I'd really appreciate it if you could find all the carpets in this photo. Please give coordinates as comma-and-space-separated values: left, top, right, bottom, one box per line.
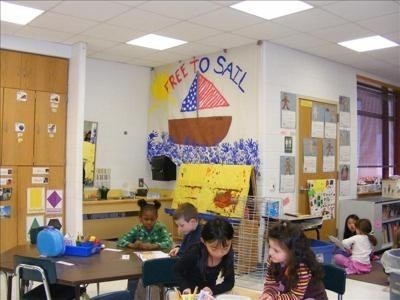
344, 252, 389, 287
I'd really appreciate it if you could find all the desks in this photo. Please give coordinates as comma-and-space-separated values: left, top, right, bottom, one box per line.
262, 213, 323, 240
0, 242, 178, 300
207, 283, 263, 300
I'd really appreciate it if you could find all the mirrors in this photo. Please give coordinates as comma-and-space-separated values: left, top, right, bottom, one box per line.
82, 120, 98, 187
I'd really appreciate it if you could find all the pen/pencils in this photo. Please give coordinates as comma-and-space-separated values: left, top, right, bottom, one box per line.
192, 286, 198, 300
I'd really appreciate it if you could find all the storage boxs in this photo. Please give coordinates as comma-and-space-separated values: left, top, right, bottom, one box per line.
309, 238, 335, 264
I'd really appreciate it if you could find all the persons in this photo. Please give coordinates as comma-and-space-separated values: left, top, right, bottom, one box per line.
171, 216, 235, 300
168, 203, 205, 257
260, 218, 329, 300
116, 198, 173, 253
343, 214, 380, 261
329, 219, 378, 275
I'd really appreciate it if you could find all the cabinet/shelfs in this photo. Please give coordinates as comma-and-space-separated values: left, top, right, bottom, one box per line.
336, 196, 400, 253
82, 196, 173, 245
0, 48, 69, 273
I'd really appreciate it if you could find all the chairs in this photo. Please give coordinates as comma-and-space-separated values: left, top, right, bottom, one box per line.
142, 257, 186, 300
11, 254, 87, 300
319, 263, 346, 300
30, 226, 60, 244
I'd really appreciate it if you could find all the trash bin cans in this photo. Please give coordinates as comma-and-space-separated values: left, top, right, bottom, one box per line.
385, 249, 400, 300
